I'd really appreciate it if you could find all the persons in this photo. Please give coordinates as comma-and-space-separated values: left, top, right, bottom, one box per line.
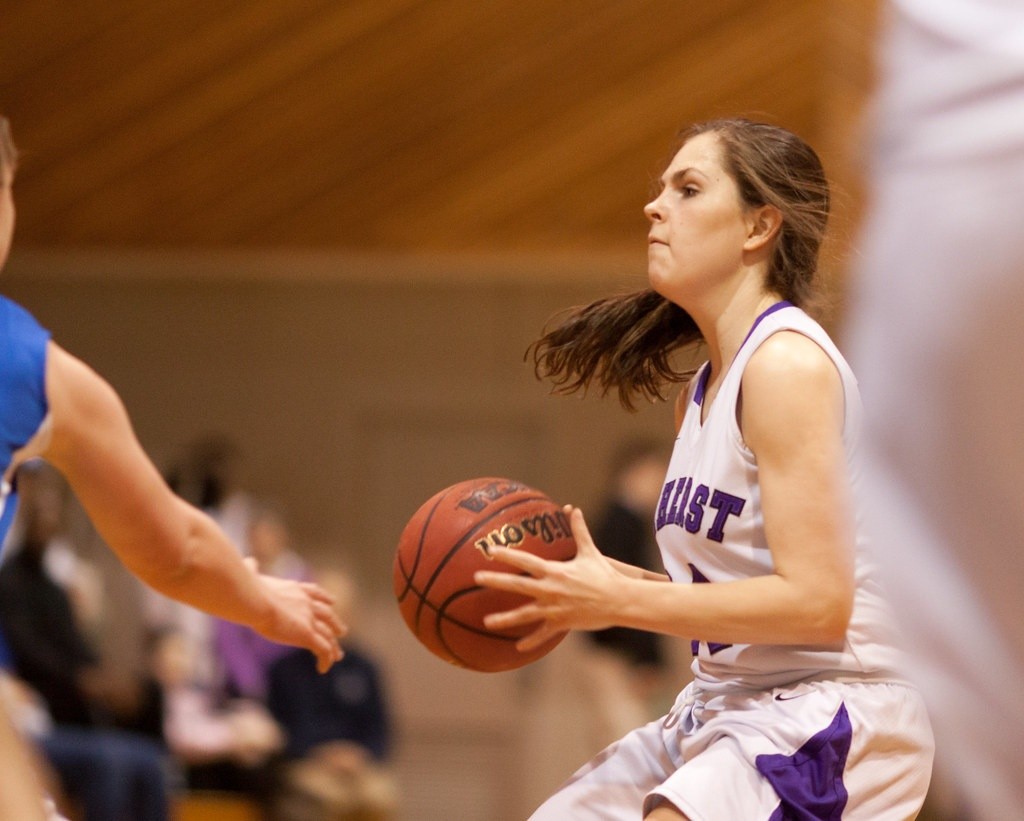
0, 111, 347, 821
0, 431, 400, 821
472, 116, 935, 821
573, 432, 672, 741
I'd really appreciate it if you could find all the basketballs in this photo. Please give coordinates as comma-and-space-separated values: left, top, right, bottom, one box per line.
392, 478, 577, 678
